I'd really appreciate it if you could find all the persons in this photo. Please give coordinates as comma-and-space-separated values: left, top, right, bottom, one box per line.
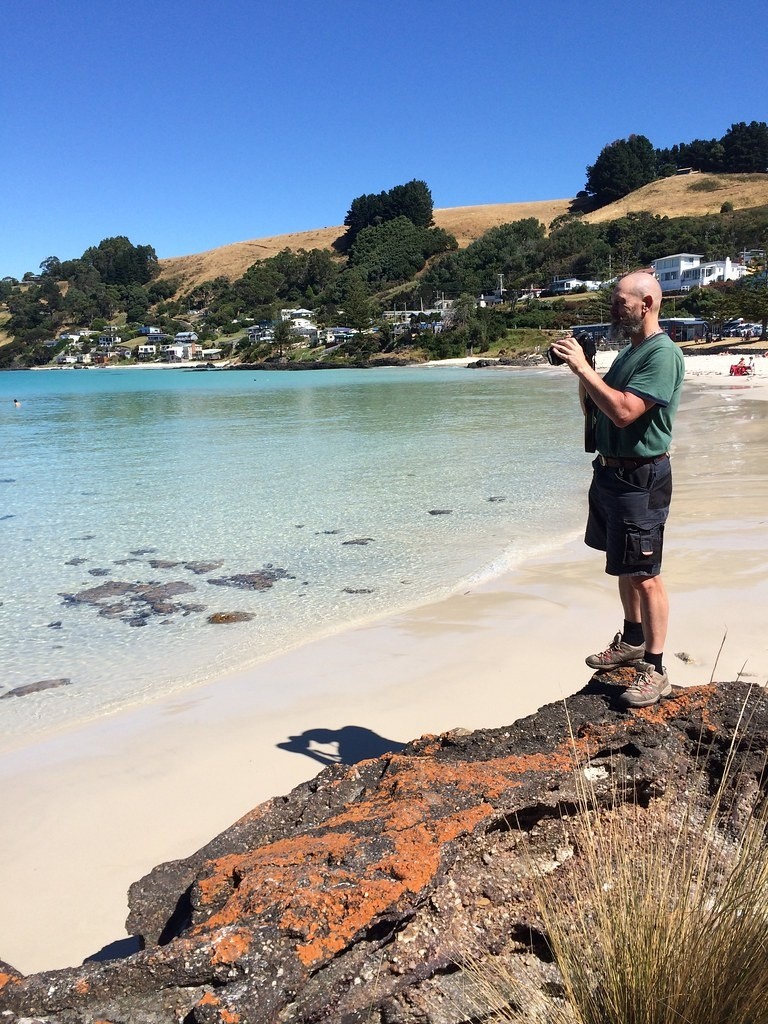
551, 273, 685, 707
730, 357, 754, 376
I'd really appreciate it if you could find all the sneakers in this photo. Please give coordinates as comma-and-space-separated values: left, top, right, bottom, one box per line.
585, 629, 647, 670
621, 661, 672, 707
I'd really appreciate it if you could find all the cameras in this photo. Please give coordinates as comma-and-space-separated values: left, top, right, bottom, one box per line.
547, 329, 597, 367
